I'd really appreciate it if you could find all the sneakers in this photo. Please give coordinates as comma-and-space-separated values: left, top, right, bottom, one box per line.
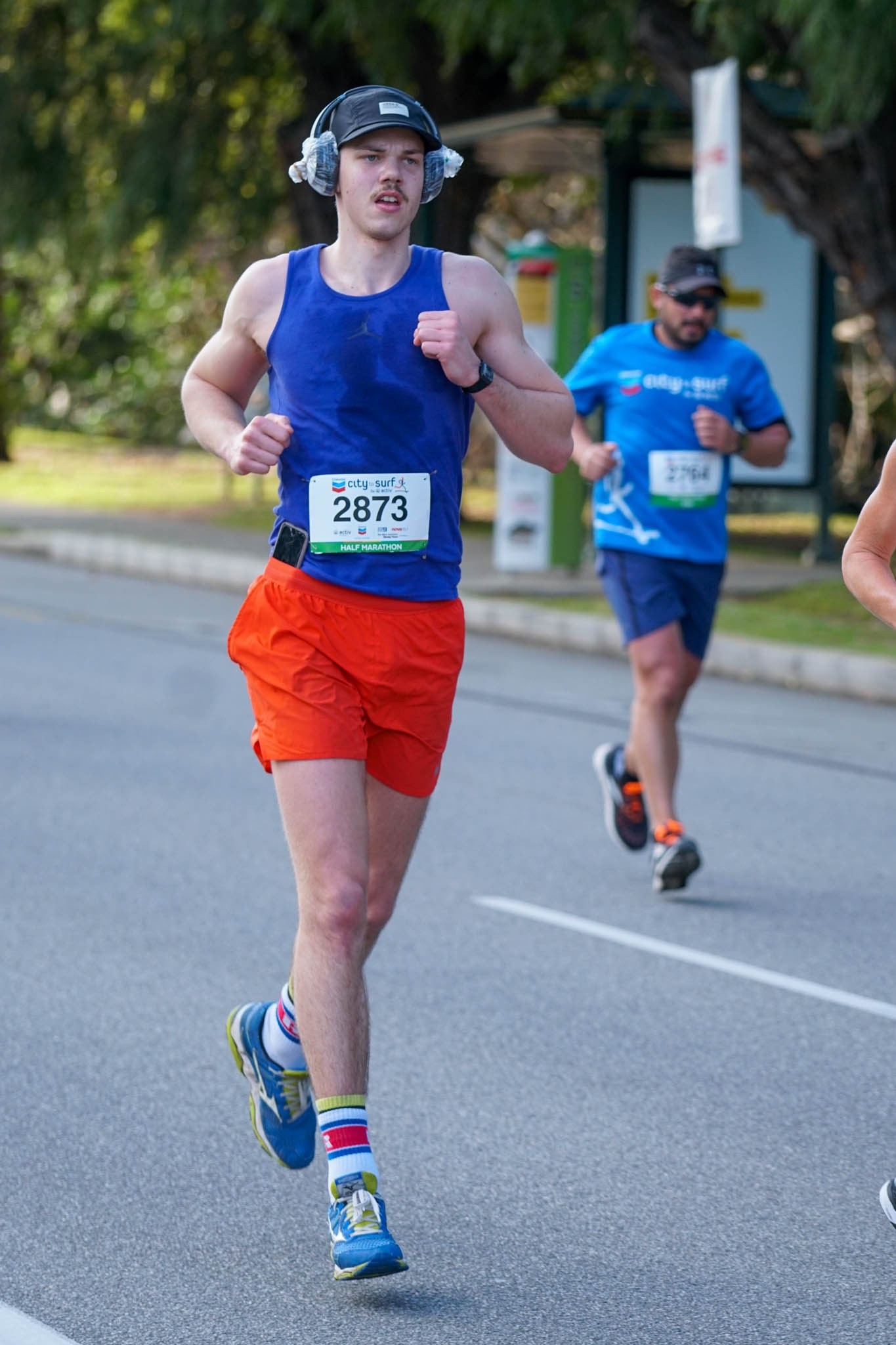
594, 740, 648, 854
647, 819, 700, 895
224, 997, 318, 1170
326, 1170, 413, 1283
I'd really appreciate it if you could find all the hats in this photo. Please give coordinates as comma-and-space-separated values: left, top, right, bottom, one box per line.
328, 89, 442, 156
661, 244, 731, 298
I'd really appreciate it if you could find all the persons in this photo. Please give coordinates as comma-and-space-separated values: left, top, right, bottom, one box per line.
842, 439, 896, 629
562, 243, 792, 893
179, 85, 578, 1286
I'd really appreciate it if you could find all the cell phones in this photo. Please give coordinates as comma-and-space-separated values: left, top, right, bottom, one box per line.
272, 521, 308, 569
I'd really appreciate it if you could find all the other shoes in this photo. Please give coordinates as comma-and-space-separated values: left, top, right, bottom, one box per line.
879, 1177, 896, 1227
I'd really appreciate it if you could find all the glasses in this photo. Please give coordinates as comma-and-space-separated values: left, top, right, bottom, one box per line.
673, 292, 717, 310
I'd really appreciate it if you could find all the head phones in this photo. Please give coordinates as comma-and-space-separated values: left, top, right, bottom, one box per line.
288, 85, 464, 204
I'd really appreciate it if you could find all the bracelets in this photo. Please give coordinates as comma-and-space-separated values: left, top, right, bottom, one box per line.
735, 432, 749, 454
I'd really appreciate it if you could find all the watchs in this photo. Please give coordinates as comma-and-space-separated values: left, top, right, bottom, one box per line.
460, 357, 494, 393
